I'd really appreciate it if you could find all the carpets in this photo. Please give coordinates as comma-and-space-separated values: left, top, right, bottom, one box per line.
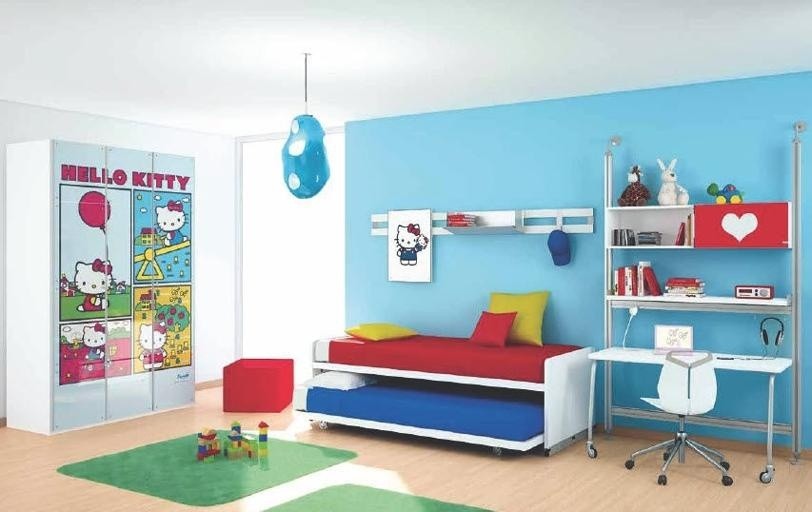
54, 424, 356, 508
260, 483, 501, 512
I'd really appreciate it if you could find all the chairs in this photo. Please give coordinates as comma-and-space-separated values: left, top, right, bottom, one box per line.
626, 348, 739, 491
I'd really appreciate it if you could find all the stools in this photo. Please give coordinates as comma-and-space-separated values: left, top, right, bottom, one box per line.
223, 359, 294, 413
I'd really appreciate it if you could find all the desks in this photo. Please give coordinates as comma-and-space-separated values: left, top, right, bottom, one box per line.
582, 344, 794, 487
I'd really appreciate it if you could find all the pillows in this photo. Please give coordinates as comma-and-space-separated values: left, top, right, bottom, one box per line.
344, 322, 422, 342
305, 373, 381, 391
489, 289, 551, 348
468, 309, 517, 347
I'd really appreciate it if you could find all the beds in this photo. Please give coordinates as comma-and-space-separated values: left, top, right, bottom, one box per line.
303, 332, 597, 459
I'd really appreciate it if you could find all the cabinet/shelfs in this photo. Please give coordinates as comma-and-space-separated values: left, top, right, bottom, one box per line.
4, 136, 201, 440
600, 115, 807, 464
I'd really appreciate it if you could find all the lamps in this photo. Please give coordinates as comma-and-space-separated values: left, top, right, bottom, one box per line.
621, 302, 642, 353
276, 49, 333, 199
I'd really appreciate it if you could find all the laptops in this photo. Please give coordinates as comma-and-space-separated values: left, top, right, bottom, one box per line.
654, 324, 693, 357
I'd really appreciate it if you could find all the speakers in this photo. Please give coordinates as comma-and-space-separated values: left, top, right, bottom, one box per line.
735, 285, 775, 299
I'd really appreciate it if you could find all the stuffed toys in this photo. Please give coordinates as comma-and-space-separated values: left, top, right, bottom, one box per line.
657, 156, 689, 205
616, 163, 653, 207
705, 181, 745, 205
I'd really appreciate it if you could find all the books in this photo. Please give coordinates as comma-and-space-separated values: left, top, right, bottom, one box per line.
612, 263, 705, 299
446, 213, 481, 229
674, 221, 685, 245
684, 214, 692, 246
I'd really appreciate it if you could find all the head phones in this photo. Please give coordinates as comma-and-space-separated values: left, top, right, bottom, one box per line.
761, 317, 784, 346
